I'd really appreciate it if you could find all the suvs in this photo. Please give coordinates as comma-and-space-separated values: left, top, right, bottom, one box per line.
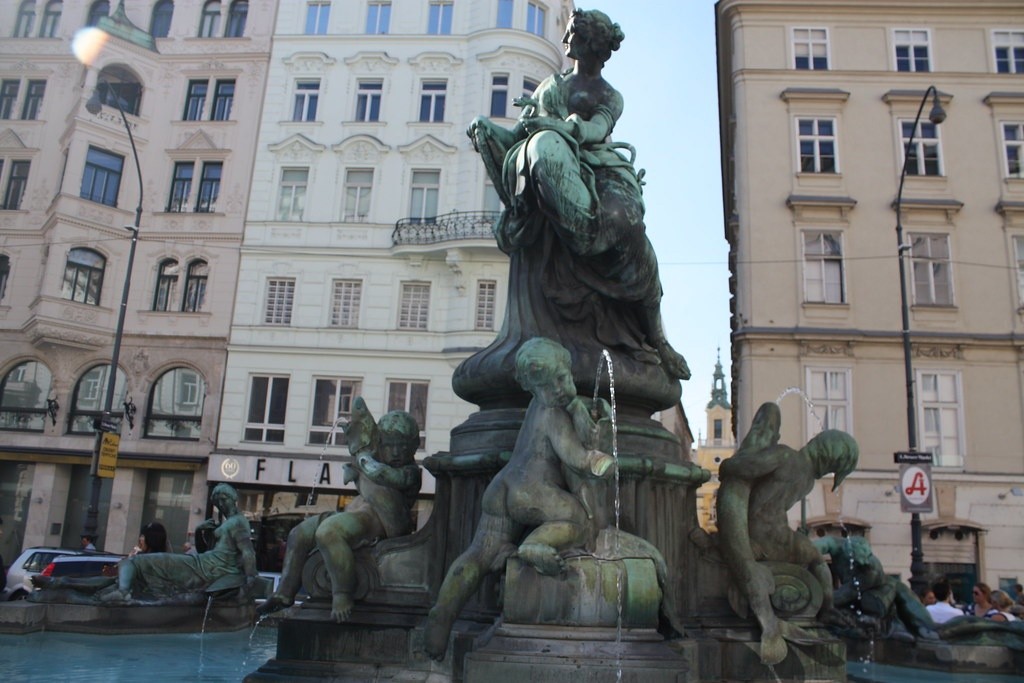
43, 555, 123, 580
1, 544, 117, 601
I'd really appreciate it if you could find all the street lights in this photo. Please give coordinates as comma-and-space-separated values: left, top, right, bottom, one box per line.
83, 72, 144, 559
894, 84, 949, 603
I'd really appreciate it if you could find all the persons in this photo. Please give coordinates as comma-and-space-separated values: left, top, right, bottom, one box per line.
135, 522, 167, 553
423, 337, 618, 659
950, 582, 998, 619
182, 542, 196, 555
1013, 584, 1024, 605
690, 428, 860, 628
467, 7, 692, 381
82, 537, 95, 549
255, 410, 422, 623
98, 485, 259, 606
920, 587, 937, 607
925, 581, 964, 623
990, 590, 1016, 621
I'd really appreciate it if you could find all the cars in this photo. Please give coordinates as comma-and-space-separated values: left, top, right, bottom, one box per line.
251, 568, 307, 609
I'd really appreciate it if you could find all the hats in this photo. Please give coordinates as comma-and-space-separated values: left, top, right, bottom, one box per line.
80, 533, 93, 539
